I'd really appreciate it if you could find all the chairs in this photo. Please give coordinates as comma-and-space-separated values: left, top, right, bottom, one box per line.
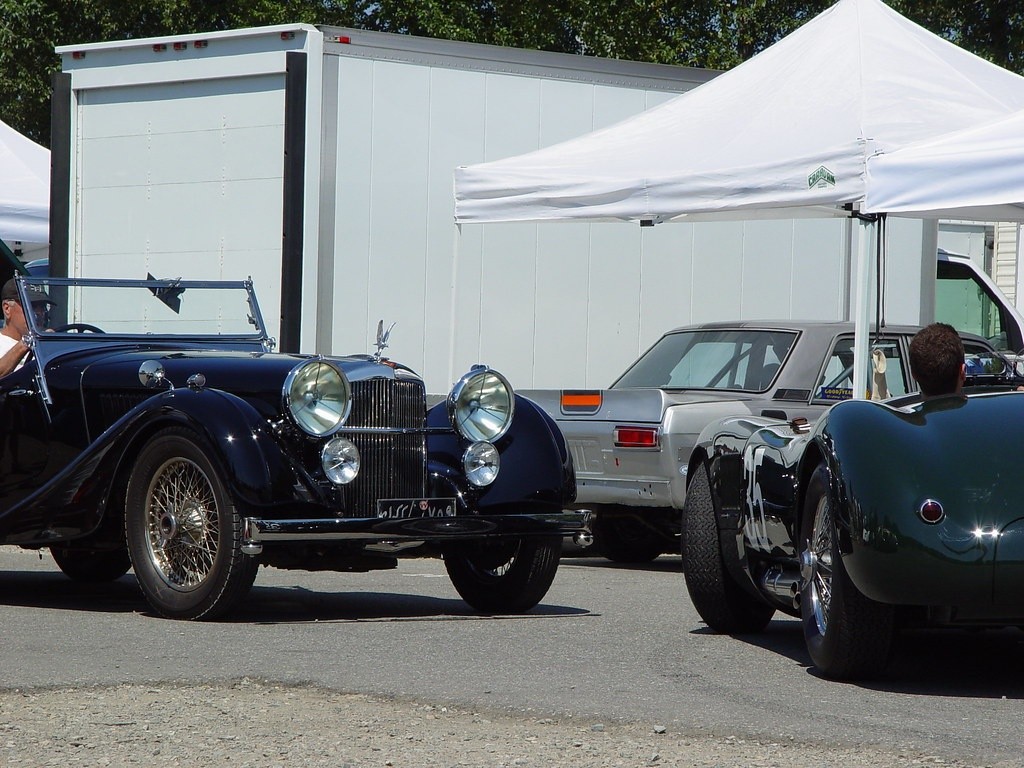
759, 364, 778, 389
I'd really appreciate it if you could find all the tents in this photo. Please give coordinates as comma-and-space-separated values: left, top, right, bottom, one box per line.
0, 120, 51, 260
450, 0, 1024, 403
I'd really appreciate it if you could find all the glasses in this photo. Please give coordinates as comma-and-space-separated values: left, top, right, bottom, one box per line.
7, 299, 55, 314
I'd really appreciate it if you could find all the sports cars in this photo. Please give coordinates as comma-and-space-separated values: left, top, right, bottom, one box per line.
678, 397, 1022, 682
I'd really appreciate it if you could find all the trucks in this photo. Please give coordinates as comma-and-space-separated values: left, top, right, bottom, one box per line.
50, 22, 1022, 394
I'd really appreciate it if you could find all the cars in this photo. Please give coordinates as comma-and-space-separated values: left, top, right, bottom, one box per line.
0, 267, 598, 625
515, 318, 1023, 567
23, 256, 49, 293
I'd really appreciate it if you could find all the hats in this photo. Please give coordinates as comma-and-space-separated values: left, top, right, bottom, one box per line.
2, 275, 58, 307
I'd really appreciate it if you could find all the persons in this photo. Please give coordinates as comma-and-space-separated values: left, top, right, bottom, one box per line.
910, 322, 966, 396
0, 279, 55, 380
